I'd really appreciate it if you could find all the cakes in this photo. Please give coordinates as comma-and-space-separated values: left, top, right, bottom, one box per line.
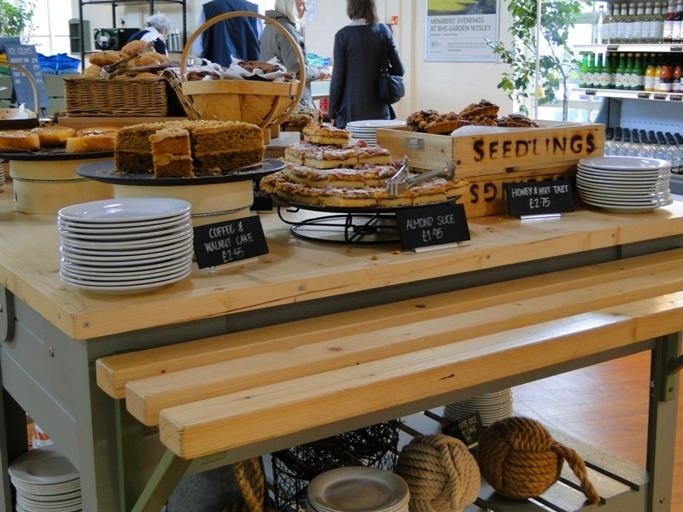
115, 121, 264, 175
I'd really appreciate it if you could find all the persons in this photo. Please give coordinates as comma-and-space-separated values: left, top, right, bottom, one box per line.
260, 0, 331, 111
327, 0, 404, 130
127, 13, 171, 57
196, 0, 262, 68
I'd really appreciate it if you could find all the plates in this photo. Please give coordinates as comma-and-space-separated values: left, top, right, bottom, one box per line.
4, 445, 82, 511
346, 119, 405, 147
0, 143, 110, 160
443, 390, 514, 428
0, 163, 5, 190
573, 154, 674, 214
54, 199, 195, 298
307, 466, 414, 511
73, 156, 288, 186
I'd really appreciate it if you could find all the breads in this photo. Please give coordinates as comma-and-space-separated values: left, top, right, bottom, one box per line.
82, 40, 291, 82
260, 124, 470, 203
407, 100, 539, 133
0, 124, 118, 158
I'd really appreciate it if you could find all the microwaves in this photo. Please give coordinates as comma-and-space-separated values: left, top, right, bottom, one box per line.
93, 27, 139, 50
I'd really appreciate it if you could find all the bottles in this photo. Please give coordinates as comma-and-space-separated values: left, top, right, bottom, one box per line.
578, 50, 683, 94
166, 28, 182, 52
604, 125, 683, 185
594, 3, 679, 43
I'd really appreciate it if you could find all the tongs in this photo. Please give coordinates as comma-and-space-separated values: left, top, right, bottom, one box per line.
387, 154, 456, 198
99, 52, 140, 80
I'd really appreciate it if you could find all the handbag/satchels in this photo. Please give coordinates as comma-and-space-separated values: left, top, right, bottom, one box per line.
374, 23, 403, 104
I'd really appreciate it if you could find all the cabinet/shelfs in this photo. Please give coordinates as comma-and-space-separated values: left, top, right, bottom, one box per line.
562, 2, 682, 201
77, 1, 189, 77
1, 125, 682, 512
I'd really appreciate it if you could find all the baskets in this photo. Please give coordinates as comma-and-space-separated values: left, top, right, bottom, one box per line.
179, 11, 304, 144
62, 76, 168, 118
